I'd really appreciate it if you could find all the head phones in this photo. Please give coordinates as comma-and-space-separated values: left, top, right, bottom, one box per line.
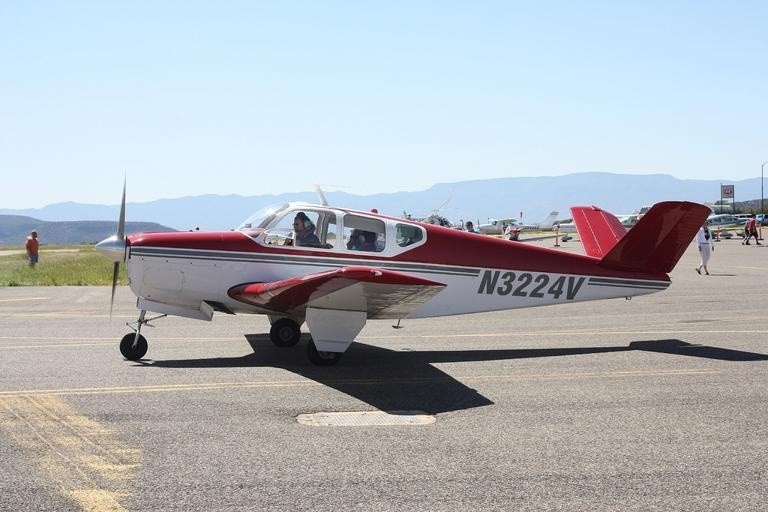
359, 234, 366, 244
296, 212, 311, 231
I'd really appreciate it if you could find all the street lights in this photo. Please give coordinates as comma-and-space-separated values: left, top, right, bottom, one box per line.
760, 159, 768, 227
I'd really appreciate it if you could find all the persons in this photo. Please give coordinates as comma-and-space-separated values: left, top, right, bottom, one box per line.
347, 228, 377, 252
466, 220, 481, 234
696, 219, 714, 275
742, 209, 762, 246
25, 229, 40, 269
284, 211, 321, 247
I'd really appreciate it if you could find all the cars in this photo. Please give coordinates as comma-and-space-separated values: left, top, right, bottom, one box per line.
755, 214, 768, 226
